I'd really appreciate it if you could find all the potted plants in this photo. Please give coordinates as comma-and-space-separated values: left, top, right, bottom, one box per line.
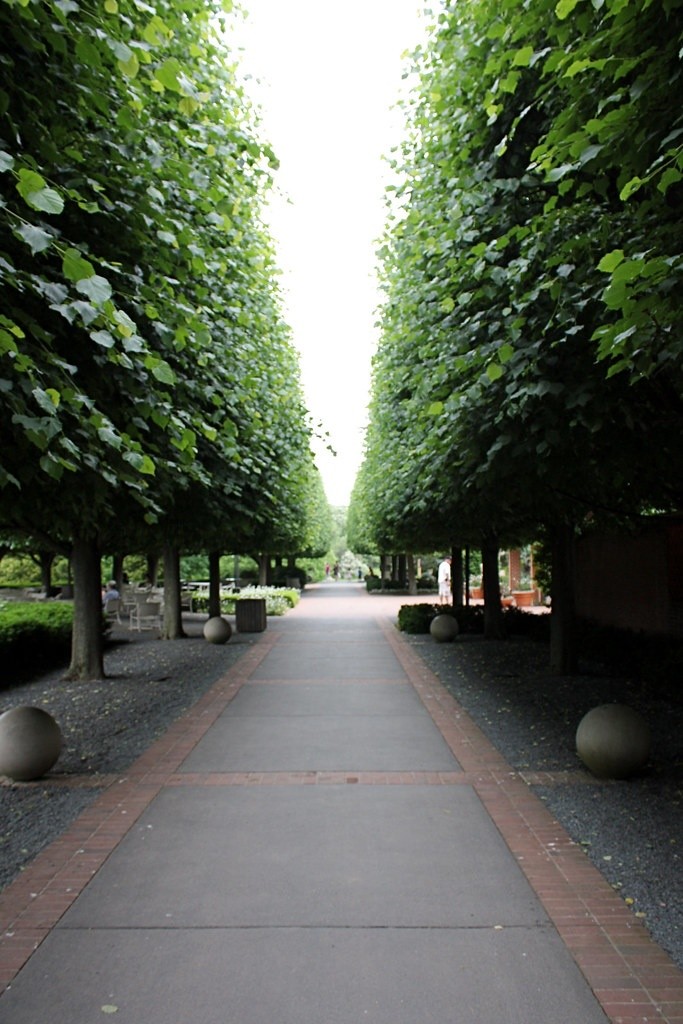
469, 574, 486, 600
511, 575, 540, 608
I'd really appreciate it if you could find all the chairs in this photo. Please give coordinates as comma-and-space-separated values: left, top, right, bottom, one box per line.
103, 584, 193, 633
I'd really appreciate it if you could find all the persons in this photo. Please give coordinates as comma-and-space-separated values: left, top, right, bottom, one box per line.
102, 581, 119, 608
334, 561, 341, 582
437, 556, 451, 604
369, 566, 373, 574
358, 566, 362, 579
345, 568, 352, 582
325, 562, 330, 578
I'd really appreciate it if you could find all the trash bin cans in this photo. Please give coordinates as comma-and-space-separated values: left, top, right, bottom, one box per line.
235, 598, 268, 633
